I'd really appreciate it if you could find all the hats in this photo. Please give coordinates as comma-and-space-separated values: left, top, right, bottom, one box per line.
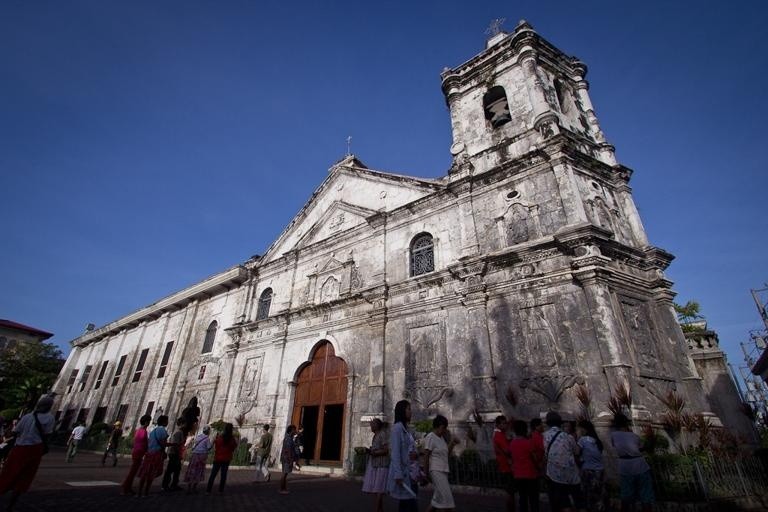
113, 420, 122, 426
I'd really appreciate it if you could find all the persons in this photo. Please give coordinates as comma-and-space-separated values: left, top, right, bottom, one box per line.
293, 425, 306, 471
0, 392, 55, 512
61, 419, 88, 464
204, 422, 237, 496
100, 420, 122, 468
251, 424, 274, 483
12, 418, 18, 430
159, 415, 188, 491
133, 414, 177, 500
354, 397, 457, 510
493, 410, 655, 511
183, 425, 217, 495
118, 414, 151, 496
276, 423, 298, 494
0, 418, 16, 465
